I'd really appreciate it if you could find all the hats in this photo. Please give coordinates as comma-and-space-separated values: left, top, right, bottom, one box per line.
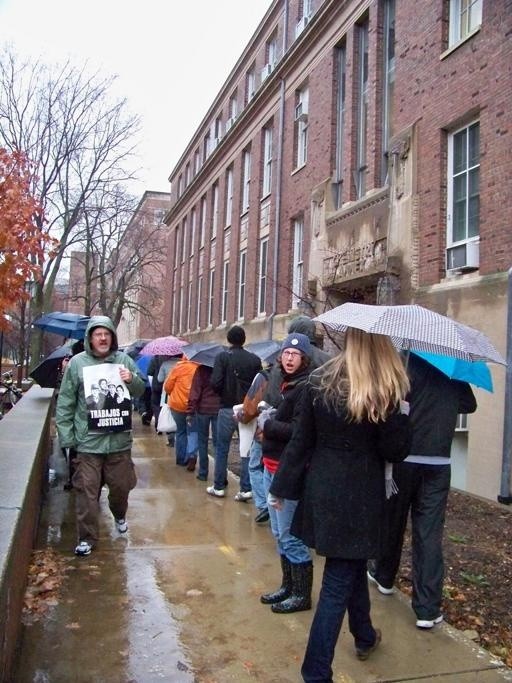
226, 325, 245, 345
280, 333, 312, 358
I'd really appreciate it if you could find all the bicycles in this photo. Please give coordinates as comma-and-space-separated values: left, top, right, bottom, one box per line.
0, 369, 22, 406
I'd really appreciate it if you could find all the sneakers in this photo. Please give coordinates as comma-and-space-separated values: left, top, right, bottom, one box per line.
166, 442, 175, 447
255, 507, 270, 523
415, 613, 444, 629
115, 518, 129, 534
206, 484, 225, 498
196, 474, 229, 488
74, 541, 94, 556
64, 480, 73, 490
234, 489, 253, 502
186, 456, 196, 472
367, 570, 393, 595
355, 629, 382, 662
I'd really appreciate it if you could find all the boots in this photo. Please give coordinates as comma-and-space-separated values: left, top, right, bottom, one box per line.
260, 553, 293, 604
271, 560, 314, 613
